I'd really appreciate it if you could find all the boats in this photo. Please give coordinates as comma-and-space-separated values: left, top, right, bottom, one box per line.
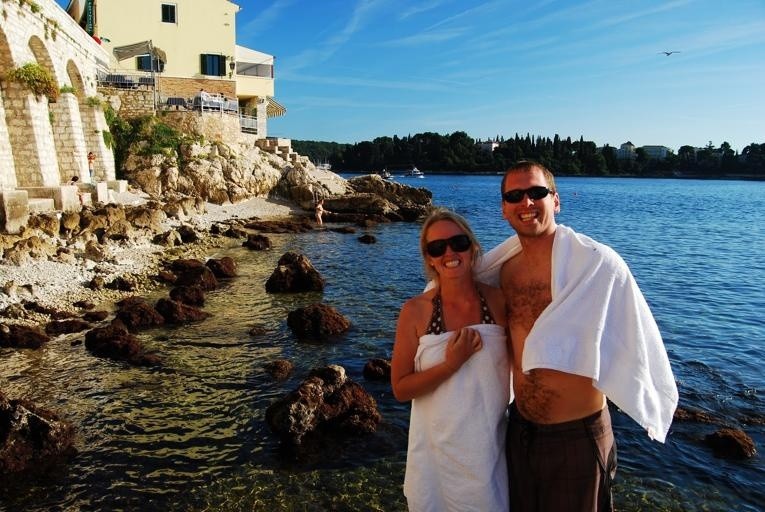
404, 167, 424, 179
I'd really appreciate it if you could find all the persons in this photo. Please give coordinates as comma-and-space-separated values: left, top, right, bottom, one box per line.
498, 161, 620, 511
390, 206, 507, 512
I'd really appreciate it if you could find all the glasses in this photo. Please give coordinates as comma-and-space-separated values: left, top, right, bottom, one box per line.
501, 185, 554, 204
426, 234, 471, 257
